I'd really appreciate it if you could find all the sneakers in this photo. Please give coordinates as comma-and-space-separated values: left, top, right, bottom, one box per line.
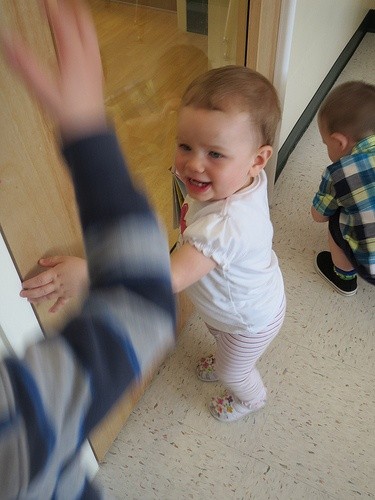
313, 248, 359, 296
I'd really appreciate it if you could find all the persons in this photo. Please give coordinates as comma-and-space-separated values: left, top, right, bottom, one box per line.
310, 80, 375, 296
0, 0, 175, 499
19, 64, 286, 423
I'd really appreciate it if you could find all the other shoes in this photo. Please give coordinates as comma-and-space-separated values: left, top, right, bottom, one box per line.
196, 351, 224, 382
208, 391, 266, 422
